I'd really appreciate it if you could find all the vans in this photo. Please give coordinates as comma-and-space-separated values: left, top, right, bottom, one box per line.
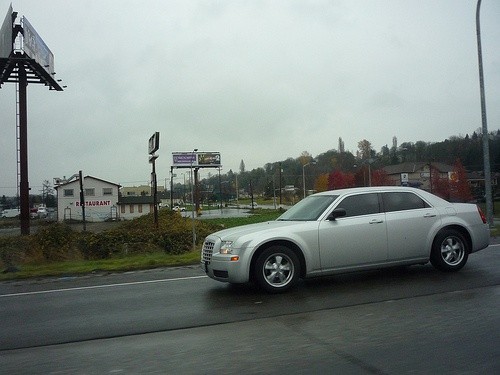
37, 207, 47, 213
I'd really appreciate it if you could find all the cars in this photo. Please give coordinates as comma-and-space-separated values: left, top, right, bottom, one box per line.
249, 202, 257, 206
200, 186, 492, 296
30, 213, 49, 220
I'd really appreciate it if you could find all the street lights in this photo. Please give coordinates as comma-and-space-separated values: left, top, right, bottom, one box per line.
303, 161, 317, 198
353, 164, 365, 186
368, 147, 409, 187
191, 148, 198, 252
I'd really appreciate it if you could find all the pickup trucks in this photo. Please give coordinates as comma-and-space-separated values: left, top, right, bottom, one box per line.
172, 206, 185, 212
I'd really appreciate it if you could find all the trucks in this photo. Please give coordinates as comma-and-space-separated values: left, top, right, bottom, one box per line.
1, 208, 20, 220
